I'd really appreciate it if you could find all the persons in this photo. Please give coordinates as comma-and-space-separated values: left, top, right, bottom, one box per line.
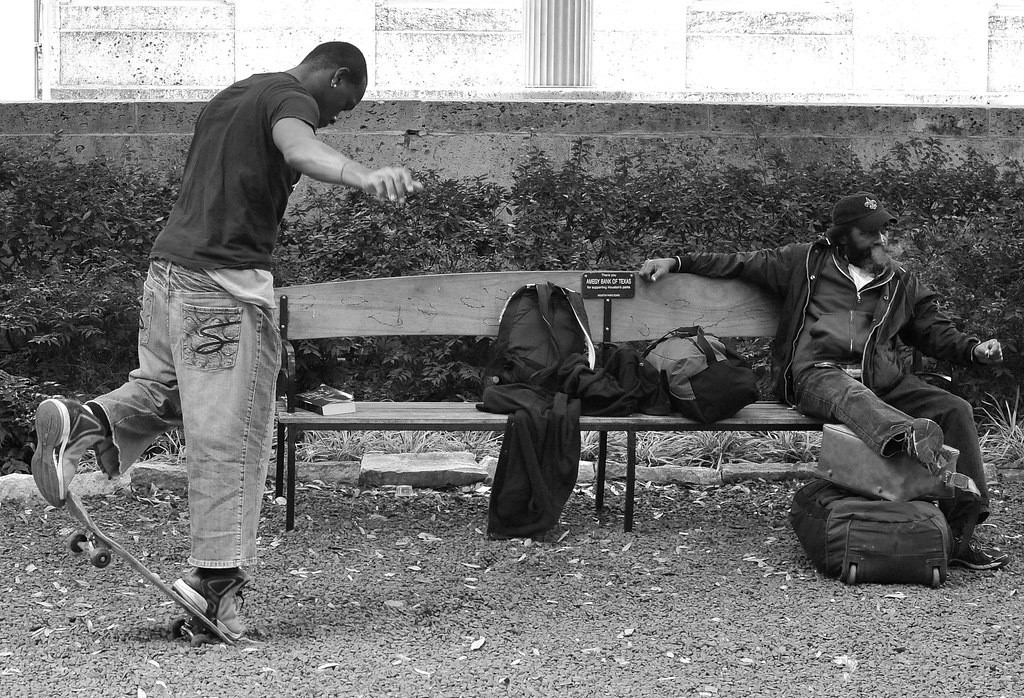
639, 191, 1010, 570
31, 42, 423, 640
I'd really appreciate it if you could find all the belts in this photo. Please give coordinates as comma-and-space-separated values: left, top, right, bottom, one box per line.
154, 257, 208, 277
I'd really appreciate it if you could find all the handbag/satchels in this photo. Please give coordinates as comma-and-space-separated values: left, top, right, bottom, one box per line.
814, 423, 983, 502
642, 325, 760, 425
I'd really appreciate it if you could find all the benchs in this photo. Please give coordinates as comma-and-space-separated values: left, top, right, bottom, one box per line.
275, 271, 838, 534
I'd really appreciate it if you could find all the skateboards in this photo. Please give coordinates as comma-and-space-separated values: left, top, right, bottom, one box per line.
64, 493, 240, 647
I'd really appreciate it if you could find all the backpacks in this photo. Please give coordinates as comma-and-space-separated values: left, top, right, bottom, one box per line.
483, 282, 595, 395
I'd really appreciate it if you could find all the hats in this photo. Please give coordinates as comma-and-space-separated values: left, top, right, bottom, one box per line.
833, 191, 898, 235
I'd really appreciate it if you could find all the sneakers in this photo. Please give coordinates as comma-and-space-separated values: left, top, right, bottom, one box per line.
890, 418, 952, 474
31, 399, 107, 507
947, 536, 1012, 570
172, 566, 251, 641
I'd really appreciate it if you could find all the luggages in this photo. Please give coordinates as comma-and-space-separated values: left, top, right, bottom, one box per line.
789, 478, 950, 588
